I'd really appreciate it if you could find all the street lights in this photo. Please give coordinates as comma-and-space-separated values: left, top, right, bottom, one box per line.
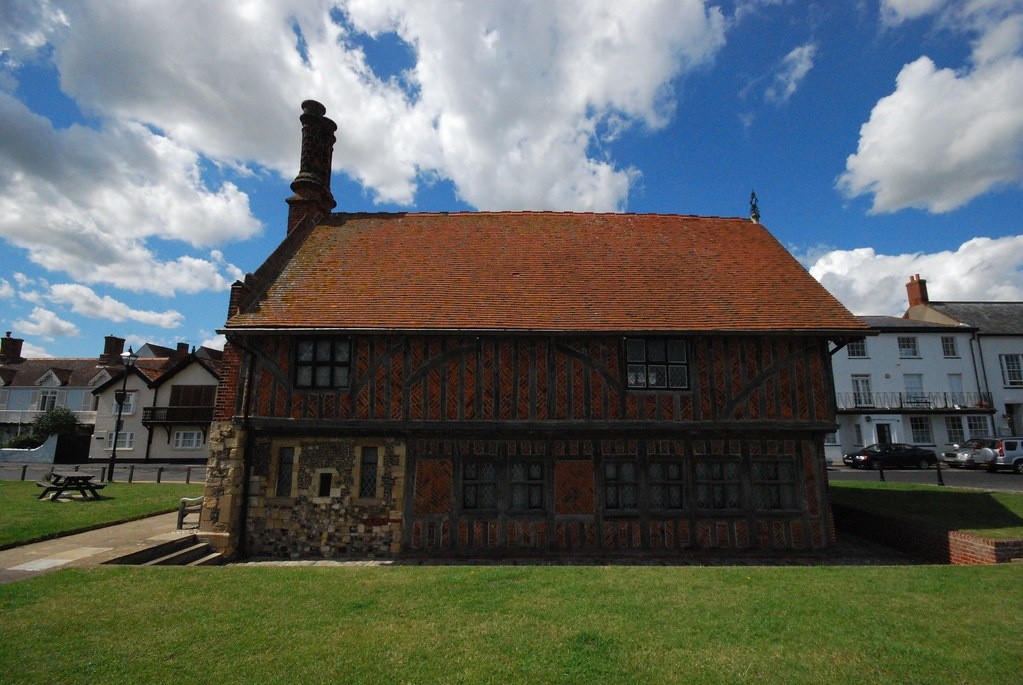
107, 344, 139, 482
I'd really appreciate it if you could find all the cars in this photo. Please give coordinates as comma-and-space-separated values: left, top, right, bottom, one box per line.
842, 442, 939, 471
941, 437, 994, 469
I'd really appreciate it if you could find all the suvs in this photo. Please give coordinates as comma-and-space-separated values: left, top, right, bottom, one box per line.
979, 436, 1023, 475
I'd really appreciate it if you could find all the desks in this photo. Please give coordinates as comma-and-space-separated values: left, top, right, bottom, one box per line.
39, 471, 100, 502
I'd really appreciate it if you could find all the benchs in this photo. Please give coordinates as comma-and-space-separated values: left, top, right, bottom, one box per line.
35, 481, 106, 501
177, 495, 205, 530
